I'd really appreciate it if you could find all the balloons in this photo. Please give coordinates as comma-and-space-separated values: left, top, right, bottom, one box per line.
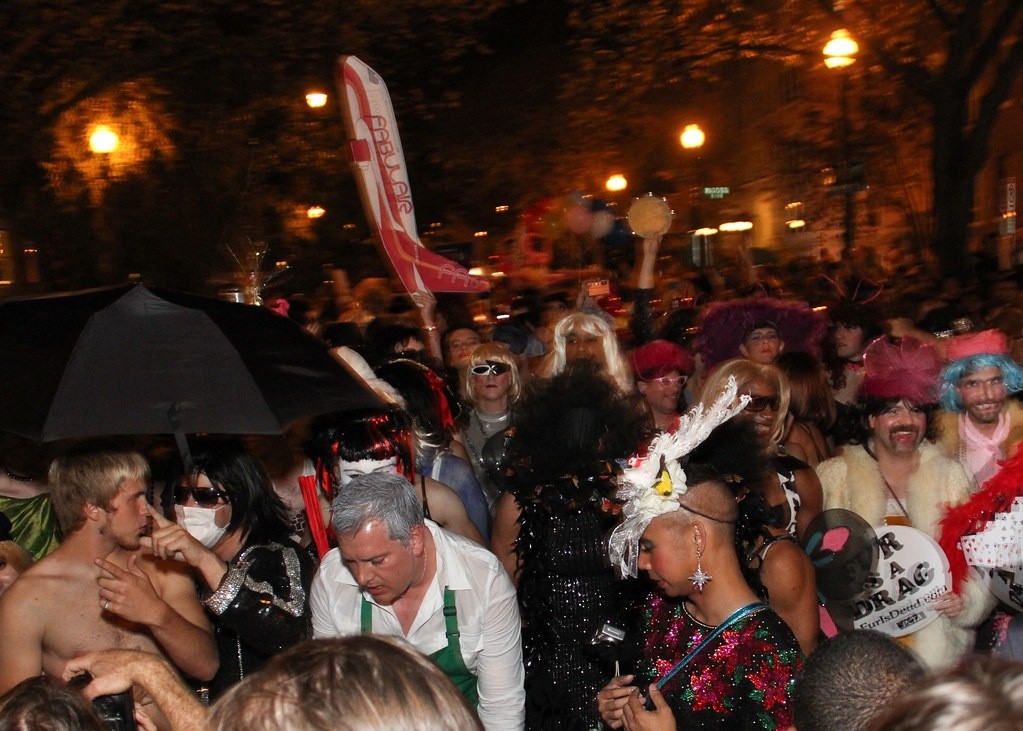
517, 191, 614, 262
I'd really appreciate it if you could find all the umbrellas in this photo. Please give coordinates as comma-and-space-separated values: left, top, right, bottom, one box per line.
0, 274, 392, 450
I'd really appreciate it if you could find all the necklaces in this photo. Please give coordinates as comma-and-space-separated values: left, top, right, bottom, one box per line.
0, 461, 34, 481
845, 359, 864, 371
409, 548, 427, 587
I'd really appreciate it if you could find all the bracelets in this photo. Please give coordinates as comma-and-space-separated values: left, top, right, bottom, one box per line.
422, 324, 439, 331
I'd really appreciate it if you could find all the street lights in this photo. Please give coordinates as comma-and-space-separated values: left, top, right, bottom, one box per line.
678, 121, 708, 302
822, 27, 859, 262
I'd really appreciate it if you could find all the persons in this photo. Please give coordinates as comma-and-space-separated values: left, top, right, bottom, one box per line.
0, 237, 1023, 731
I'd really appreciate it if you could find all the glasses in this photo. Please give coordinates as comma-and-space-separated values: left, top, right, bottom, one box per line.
650, 376, 688, 387
735, 393, 780, 413
470, 365, 511, 375
173, 487, 228, 508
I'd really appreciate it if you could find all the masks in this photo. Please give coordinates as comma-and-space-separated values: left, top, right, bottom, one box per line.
172, 503, 234, 550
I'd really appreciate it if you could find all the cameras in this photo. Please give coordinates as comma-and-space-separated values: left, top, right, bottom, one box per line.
590, 623, 625, 653
67, 674, 138, 731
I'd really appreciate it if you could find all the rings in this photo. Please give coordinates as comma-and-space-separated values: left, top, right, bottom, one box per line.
103, 601, 110, 608
